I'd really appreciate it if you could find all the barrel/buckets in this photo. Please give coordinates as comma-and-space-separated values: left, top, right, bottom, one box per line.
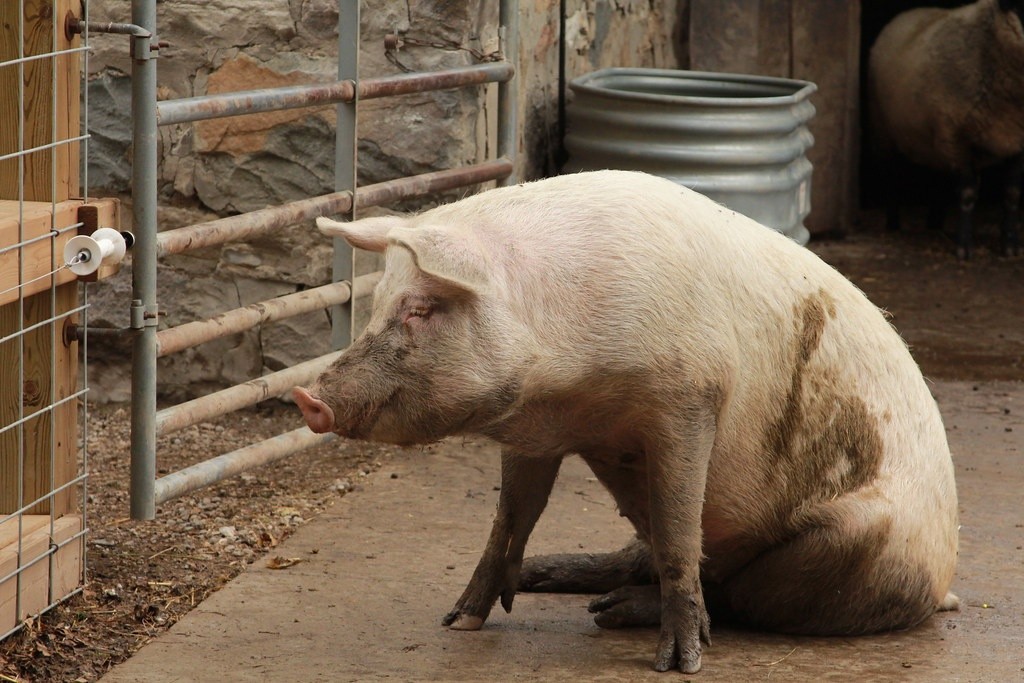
566, 67, 817, 249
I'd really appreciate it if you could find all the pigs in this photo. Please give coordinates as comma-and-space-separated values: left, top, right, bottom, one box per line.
289, 169, 963, 675
867, 0, 1024, 210
